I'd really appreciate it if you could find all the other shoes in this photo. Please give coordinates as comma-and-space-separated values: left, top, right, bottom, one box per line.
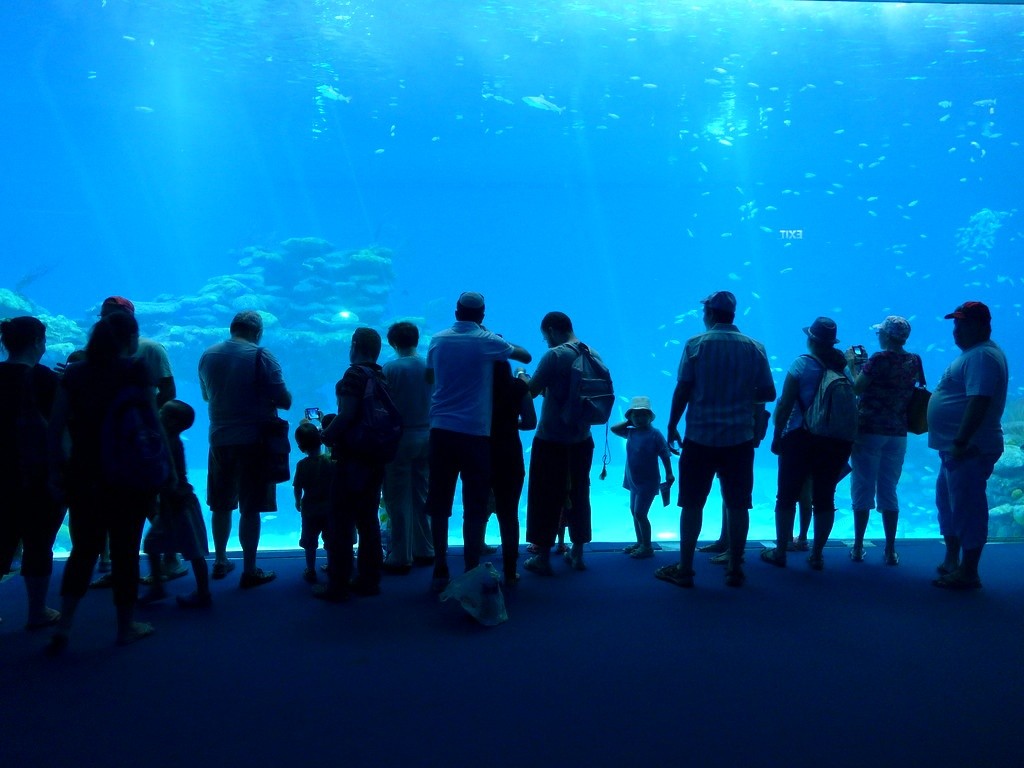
807, 552, 824, 572
761, 548, 786, 567
882, 548, 900, 565
934, 569, 983, 589
936, 559, 961, 574
850, 547, 866, 562
785, 537, 809, 552
27, 539, 748, 650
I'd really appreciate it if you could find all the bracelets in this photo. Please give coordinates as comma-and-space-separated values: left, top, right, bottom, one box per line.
515, 371, 525, 378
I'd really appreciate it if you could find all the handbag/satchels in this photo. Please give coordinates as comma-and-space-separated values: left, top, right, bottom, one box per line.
253, 415, 291, 484
907, 354, 932, 435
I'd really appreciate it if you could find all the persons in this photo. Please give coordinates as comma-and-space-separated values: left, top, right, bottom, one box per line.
293, 329, 387, 598
381, 323, 435, 574
0, 316, 69, 632
844, 316, 931, 565
517, 312, 594, 574
655, 292, 853, 588
611, 407, 674, 558
423, 292, 536, 592
927, 301, 1009, 587
198, 312, 291, 590
56, 297, 214, 640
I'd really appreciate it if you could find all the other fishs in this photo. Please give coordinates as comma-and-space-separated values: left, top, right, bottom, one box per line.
83, 0, 1024, 527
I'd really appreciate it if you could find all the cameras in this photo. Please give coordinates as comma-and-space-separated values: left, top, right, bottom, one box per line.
852, 345, 863, 357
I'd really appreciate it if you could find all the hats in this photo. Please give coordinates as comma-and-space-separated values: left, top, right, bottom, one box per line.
625, 397, 656, 423
700, 290, 737, 313
458, 291, 484, 309
802, 317, 841, 344
102, 295, 134, 317
873, 316, 911, 341
945, 301, 991, 324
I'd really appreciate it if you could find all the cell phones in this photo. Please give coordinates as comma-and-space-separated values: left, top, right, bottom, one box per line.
306, 408, 320, 420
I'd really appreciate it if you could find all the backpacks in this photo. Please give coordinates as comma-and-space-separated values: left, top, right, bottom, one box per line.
795, 354, 859, 447
546, 342, 615, 426
353, 365, 405, 450
100, 356, 177, 495
